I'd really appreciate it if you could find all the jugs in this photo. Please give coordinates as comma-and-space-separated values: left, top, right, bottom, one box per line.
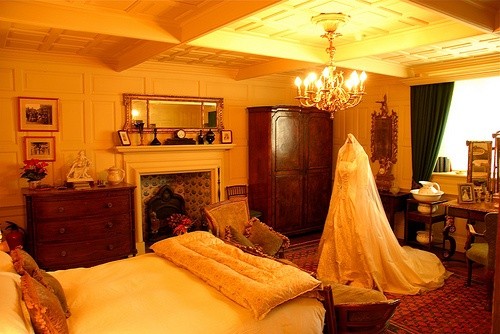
106, 166, 125, 184
418, 181, 440, 195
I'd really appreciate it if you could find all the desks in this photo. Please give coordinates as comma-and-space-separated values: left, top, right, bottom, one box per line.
377, 192, 411, 246
440, 199, 500, 265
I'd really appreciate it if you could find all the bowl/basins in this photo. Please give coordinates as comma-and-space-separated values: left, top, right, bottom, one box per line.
410, 189, 444, 201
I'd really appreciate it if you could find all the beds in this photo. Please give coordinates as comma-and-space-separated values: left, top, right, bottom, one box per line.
0, 245, 337, 334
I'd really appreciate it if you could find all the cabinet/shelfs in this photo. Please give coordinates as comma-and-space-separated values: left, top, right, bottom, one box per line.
404, 198, 448, 256
246, 103, 333, 237
22, 184, 138, 271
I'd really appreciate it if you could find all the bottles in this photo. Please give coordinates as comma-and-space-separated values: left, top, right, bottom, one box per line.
416, 231, 433, 242
151, 128, 161, 145
198, 130, 204, 144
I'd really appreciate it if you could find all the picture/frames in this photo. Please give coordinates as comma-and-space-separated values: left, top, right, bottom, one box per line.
457, 182, 475, 204
117, 130, 132, 146
221, 129, 233, 144
23, 135, 57, 162
17, 96, 60, 132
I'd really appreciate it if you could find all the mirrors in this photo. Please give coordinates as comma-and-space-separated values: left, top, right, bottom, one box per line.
121, 93, 226, 131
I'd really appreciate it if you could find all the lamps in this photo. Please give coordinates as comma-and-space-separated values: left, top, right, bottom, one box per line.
292, 11, 369, 118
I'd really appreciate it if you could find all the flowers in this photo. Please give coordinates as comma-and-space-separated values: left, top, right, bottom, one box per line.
18, 158, 49, 180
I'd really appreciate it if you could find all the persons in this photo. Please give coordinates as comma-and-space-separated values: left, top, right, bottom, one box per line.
316, 134, 455, 294
28, 112, 47, 121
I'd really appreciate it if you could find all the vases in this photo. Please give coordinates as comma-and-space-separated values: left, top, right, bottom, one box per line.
29, 179, 43, 190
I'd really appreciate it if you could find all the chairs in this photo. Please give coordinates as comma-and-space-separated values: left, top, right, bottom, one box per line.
200, 196, 292, 260
226, 184, 263, 220
462, 212, 498, 311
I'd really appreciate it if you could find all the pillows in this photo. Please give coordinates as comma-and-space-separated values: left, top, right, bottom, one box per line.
21, 271, 69, 334
150, 230, 323, 322
12, 245, 39, 277
224, 216, 290, 258
33, 266, 71, 319
